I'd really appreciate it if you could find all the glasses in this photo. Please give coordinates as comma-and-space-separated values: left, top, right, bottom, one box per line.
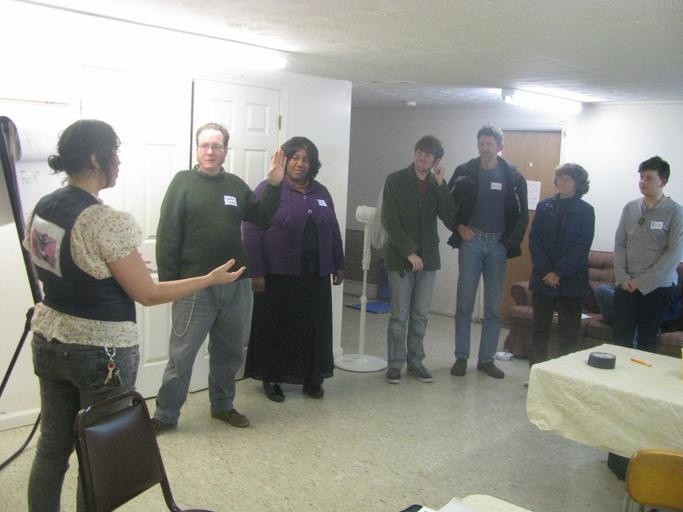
199, 143, 222, 153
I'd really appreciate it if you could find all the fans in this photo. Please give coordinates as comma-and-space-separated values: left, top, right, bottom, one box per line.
333, 186, 391, 371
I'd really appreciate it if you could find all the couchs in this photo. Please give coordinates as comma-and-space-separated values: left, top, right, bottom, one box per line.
504, 250, 682, 362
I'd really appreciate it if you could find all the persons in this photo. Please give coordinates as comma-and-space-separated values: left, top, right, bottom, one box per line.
379, 135, 456, 385
522, 161, 596, 390
611, 156, 682, 352
446, 123, 530, 380
149, 122, 288, 435
242, 136, 345, 403
21, 119, 248, 512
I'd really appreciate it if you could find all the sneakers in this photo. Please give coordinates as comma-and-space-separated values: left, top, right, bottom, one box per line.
385, 368, 402, 384
406, 365, 433, 382
451, 358, 467, 376
211, 409, 250, 428
477, 361, 504, 378
151, 418, 178, 436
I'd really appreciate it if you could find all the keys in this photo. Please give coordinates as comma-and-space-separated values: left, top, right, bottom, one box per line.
104, 362, 123, 385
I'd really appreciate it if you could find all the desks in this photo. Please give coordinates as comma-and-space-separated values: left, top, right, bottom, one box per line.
525, 341, 681, 479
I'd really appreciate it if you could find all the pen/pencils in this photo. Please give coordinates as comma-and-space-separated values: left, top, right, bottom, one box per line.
631, 358, 652, 367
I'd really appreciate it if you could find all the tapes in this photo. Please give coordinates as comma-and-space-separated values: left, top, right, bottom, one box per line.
587, 352, 616, 369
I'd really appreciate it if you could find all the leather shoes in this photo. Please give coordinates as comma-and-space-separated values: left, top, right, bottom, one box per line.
303, 383, 325, 399
263, 379, 284, 402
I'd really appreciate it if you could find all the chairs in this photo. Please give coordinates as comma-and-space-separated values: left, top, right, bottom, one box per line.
622, 449, 682, 512
70, 390, 210, 512
